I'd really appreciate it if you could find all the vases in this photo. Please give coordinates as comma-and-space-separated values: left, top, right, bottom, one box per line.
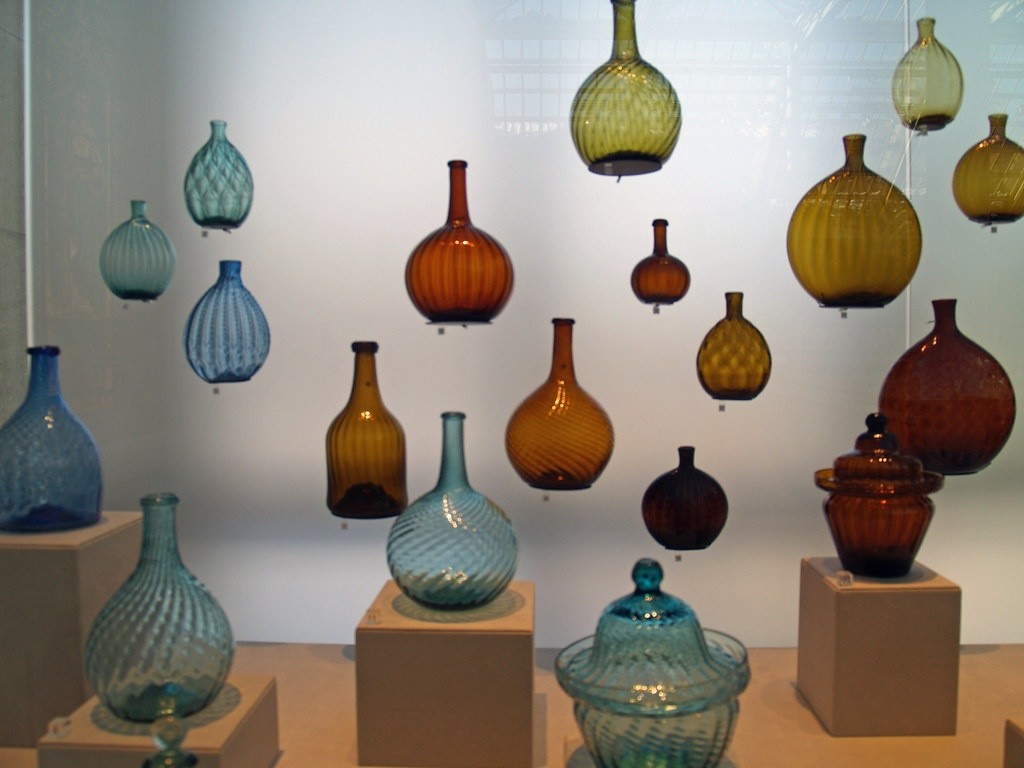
1, 1, 1024, 724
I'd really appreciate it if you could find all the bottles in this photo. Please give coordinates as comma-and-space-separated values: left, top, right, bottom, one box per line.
386, 410, 520, 613
631, 219, 690, 305
0, 344, 103, 533
695, 291, 772, 400
325, 342, 408, 520
505, 317, 615, 491
787, 16, 1024, 475
568, 0, 683, 176
406, 160, 515, 324
100, 119, 271, 384
641, 447, 728, 550
85, 490, 233, 768
